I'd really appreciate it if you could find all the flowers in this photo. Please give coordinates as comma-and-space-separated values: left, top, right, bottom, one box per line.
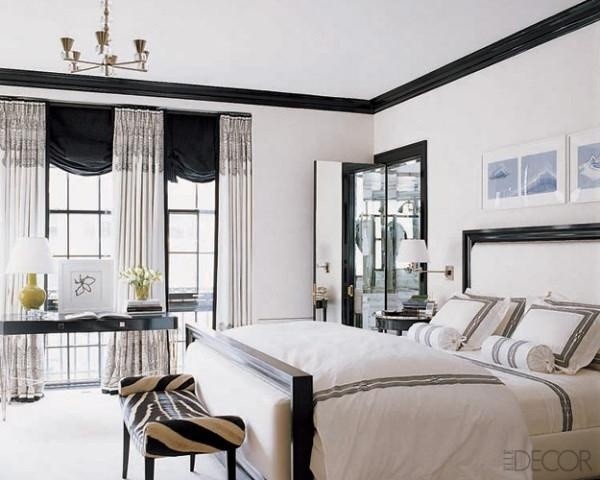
118, 264, 164, 285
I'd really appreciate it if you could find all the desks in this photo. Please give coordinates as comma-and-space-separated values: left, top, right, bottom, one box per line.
314, 298, 327, 322
373, 305, 434, 337
0, 312, 180, 423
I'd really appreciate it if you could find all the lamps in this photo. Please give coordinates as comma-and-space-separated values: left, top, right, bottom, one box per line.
397, 201, 419, 214
4, 236, 60, 311
58, 0, 152, 77
395, 237, 455, 282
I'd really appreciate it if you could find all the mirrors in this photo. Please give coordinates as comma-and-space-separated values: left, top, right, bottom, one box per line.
374, 140, 426, 297
312, 159, 386, 334
340, 161, 385, 333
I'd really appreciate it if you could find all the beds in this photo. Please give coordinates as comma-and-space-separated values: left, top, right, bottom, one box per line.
181, 221, 599, 479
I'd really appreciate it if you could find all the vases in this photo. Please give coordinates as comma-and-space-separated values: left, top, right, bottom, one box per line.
129, 284, 152, 301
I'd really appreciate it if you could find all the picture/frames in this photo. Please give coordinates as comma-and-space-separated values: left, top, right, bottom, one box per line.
57, 258, 115, 315
569, 127, 600, 204
482, 133, 568, 212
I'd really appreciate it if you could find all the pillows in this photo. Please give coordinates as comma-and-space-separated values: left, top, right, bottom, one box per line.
480, 335, 555, 373
463, 287, 527, 338
428, 291, 511, 351
406, 322, 464, 352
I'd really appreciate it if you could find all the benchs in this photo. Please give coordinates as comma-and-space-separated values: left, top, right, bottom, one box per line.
117, 372, 246, 480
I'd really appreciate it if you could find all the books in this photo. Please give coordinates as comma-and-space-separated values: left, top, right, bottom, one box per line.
379, 294, 437, 317
123, 299, 165, 318
63, 311, 131, 321
